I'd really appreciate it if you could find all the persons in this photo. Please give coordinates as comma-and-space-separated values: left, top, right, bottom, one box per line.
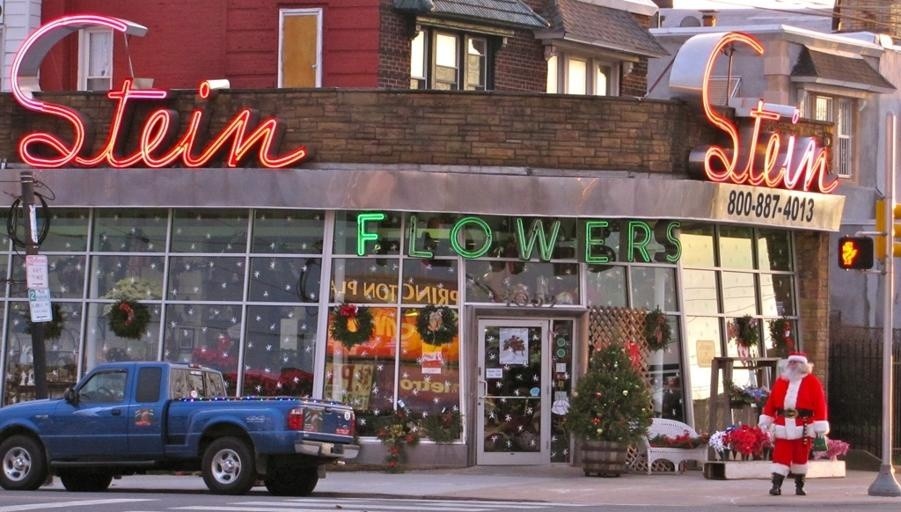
759, 350, 831, 496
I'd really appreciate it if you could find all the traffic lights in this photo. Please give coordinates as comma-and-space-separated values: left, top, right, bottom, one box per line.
837, 234, 873, 272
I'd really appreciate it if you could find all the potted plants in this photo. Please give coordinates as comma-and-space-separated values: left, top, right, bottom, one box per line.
565, 343, 653, 478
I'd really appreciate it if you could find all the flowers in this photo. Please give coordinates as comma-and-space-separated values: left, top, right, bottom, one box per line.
708, 424, 851, 461
729, 384, 771, 406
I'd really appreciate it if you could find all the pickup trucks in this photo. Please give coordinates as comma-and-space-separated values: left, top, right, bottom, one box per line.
0, 361, 360, 495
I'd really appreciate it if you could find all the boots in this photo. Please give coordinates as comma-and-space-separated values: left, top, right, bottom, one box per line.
770, 471, 785, 496
791, 473, 806, 495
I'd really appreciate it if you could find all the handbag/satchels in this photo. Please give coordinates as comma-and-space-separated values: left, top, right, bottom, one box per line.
813, 433, 827, 450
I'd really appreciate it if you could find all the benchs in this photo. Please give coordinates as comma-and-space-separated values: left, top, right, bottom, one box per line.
625, 418, 708, 474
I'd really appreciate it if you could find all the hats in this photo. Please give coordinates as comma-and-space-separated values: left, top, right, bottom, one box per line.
788, 351, 807, 363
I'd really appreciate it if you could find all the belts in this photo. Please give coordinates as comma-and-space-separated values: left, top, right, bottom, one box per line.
776, 409, 814, 417
